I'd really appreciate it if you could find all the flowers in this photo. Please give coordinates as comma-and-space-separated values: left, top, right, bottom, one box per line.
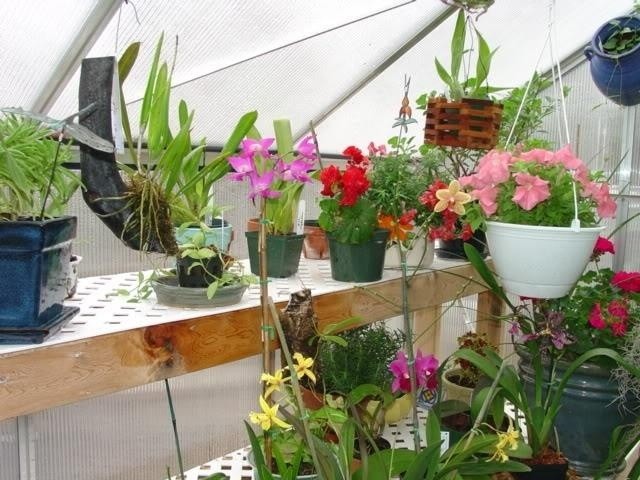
225, 133, 639, 479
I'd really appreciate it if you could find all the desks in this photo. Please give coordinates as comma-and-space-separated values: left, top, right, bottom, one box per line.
0, 252, 504, 479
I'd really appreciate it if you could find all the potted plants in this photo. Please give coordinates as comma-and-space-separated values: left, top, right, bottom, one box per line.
584, 16, 640, 107
418, 11, 502, 151
0, 30, 258, 345
